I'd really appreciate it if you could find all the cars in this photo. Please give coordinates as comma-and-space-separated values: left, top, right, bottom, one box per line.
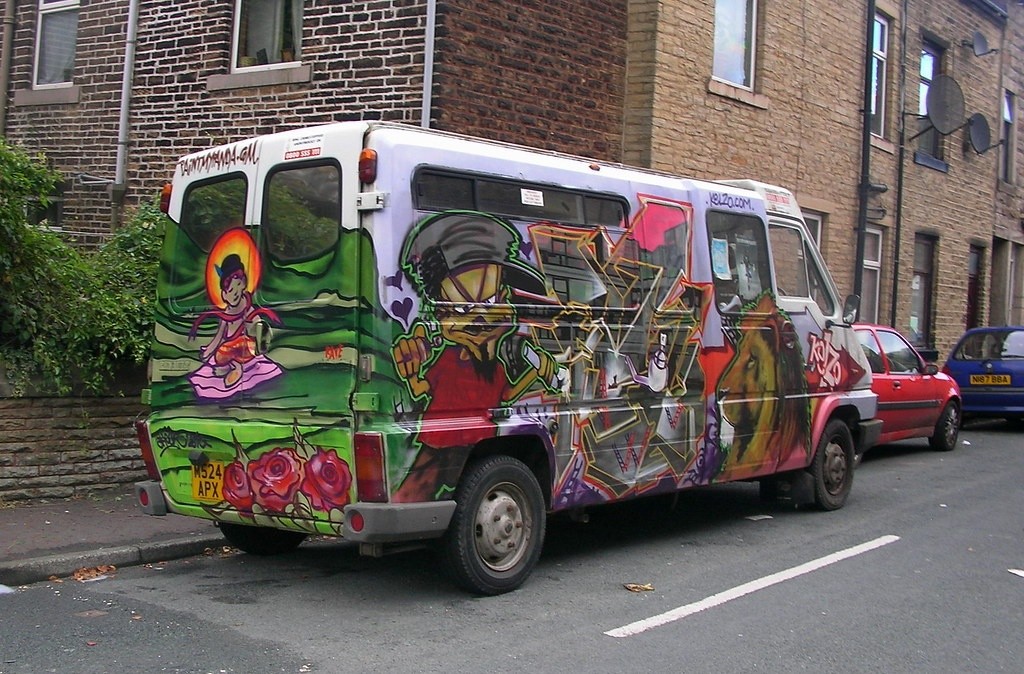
848, 322, 962, 466
942, 326, 1024, 430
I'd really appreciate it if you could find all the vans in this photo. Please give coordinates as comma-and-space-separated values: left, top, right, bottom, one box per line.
132, 120, 887, 593
